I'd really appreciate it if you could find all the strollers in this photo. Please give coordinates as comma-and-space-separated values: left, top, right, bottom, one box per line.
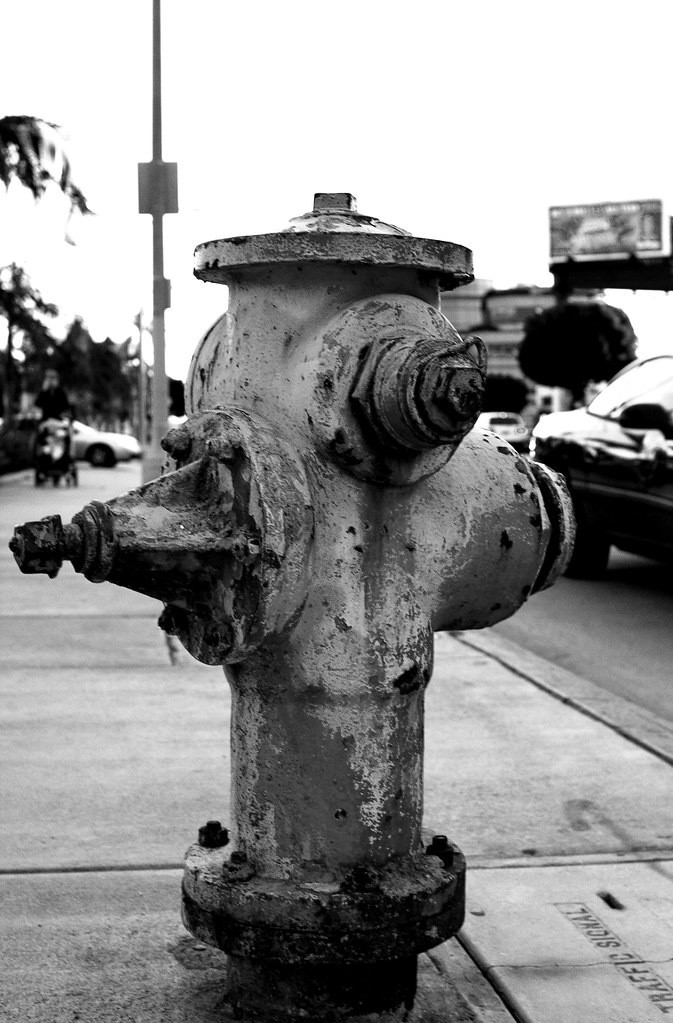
32, 417, 78, 490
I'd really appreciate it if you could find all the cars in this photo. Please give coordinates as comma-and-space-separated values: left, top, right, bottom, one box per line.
527, 351, 673, 585
28, 409, 143, 469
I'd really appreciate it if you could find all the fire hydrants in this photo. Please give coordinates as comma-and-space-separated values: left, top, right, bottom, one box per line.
7, 192, 577, 1023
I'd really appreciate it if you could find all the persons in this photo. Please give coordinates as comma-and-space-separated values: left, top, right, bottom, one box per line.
534, 397, 551, 426
34, 371, 69, 482
